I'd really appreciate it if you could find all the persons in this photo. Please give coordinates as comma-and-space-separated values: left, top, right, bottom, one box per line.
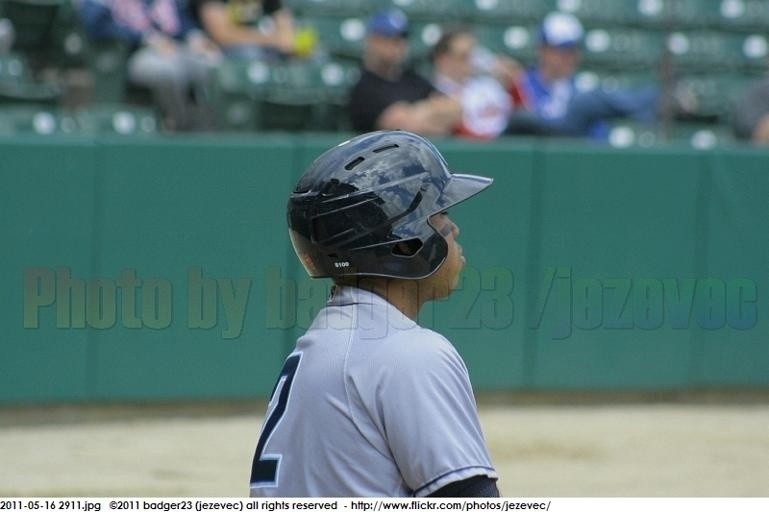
247, 128, 494, 496
350, 6, 767, 148
1, 0, 332, 131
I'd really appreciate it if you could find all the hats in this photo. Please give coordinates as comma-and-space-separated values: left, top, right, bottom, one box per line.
365, 8, 411, 37
537, 9, 586, 47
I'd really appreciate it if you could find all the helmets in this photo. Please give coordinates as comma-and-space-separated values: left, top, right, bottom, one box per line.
286, 130, 494, 282
